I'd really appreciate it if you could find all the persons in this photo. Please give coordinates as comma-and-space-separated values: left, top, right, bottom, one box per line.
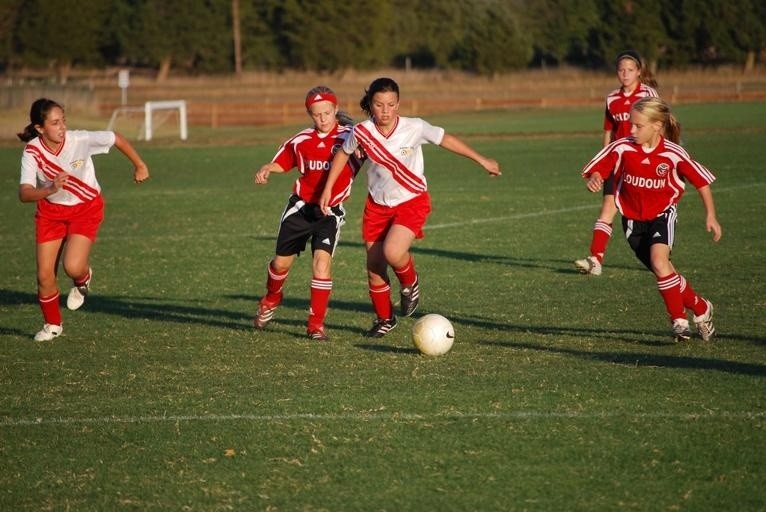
579, 96, 722, 343
17, 97, 150, 342
319, 78, 502, 339
254, 86, 368, 341
574, 52, 663, 277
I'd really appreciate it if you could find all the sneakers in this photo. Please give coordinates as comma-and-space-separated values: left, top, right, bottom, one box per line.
308, 329, 329, 340
673, 319, 692, 344
400, 272, 419, 317
34, 323, 63, 341
365, 313, 396, 339
67, 268, 92, 310
574, 257, 602, 277
693, 297, 716, 342
254, 289, 283, 329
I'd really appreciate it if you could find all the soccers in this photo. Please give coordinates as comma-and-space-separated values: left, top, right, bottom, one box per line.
412, 313, 455, 355
